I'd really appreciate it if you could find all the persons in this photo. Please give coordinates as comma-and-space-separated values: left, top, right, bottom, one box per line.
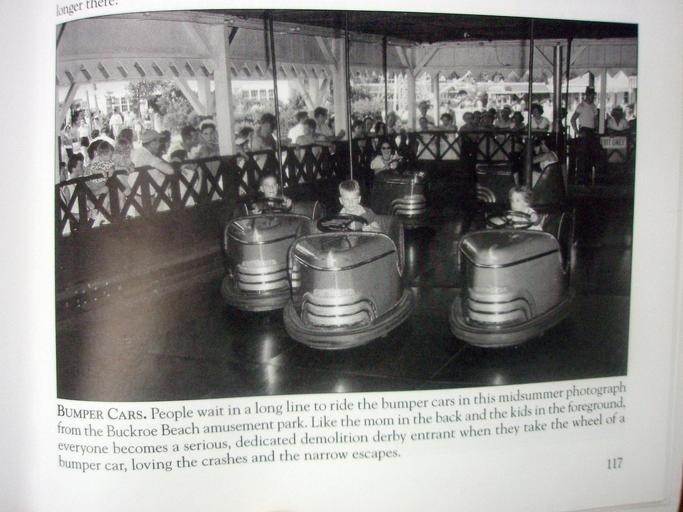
337, 179, 381, 233
351, 100, 462, 173
484, 187, 544, 233
250, 176, 292, 214
237, 106, 345, 179
458, 103, 558, 171
571, 87, 634, 137
60, 90, 219, 229
